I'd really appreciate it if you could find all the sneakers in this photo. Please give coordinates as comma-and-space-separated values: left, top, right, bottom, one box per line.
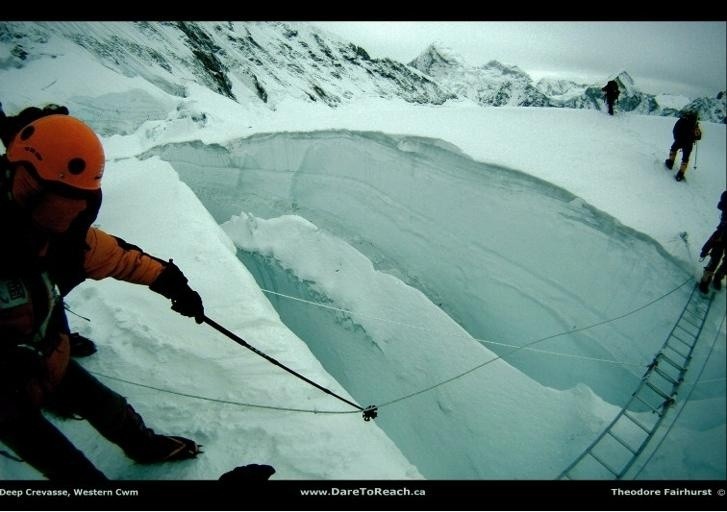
699, 278, 723, 294
665, 158, 685, 181
134, 434, 201, 462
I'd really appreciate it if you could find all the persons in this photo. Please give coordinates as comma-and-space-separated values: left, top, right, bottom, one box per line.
696, 188, 725, 294
602, 80, 621, 115
0, 113, 204, 482
665, 111, 702, 182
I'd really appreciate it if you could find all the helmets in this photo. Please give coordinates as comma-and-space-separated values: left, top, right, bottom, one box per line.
6, 114, 104, 191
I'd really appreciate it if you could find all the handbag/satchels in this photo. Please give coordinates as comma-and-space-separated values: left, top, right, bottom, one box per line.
695, 128, 702, 140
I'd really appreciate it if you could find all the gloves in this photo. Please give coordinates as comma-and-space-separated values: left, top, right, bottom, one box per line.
150, 261, 207, 325
